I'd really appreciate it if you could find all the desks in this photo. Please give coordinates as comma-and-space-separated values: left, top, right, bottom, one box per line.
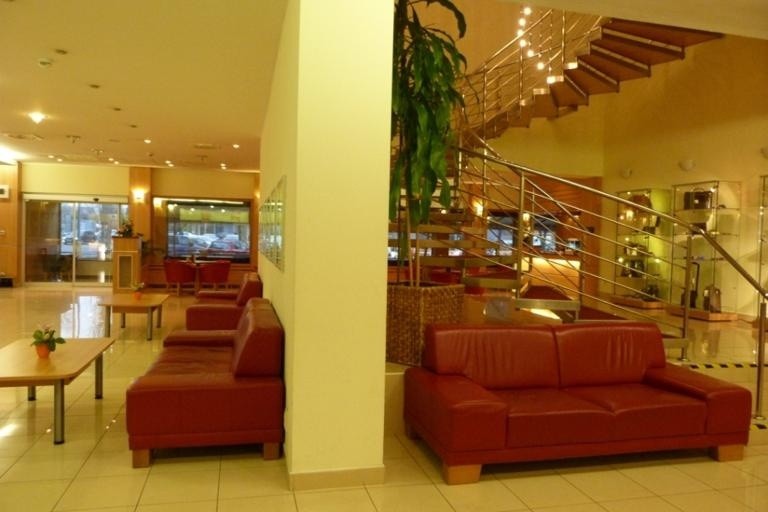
1, 337, 116, 447
96, 293, 170, 341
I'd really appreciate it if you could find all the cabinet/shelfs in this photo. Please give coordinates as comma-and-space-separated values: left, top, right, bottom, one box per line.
523, 170, 744, 322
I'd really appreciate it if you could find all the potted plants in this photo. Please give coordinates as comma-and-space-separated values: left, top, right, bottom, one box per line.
29, 329, 66, 359
387, 2, 468, 369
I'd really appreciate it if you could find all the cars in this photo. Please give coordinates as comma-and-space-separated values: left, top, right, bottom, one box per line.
63, 228, 250, 256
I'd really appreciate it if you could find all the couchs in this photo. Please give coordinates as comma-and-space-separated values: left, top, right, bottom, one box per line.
404, 316, 754, 487
125, 296, 283, 468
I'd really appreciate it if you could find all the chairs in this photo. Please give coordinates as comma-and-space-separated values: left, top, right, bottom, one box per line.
163, 256, 232, 297
185, 270, 263, 330
